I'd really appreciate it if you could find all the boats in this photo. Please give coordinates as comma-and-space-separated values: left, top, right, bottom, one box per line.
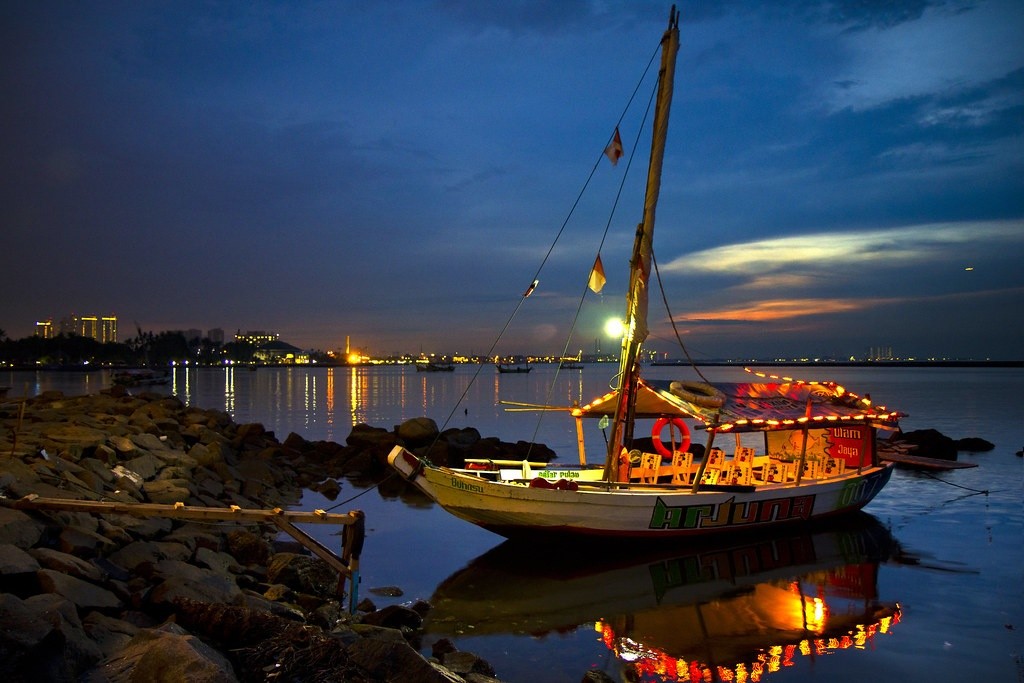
413, 363, 426, 373
492, 360, 533, 376
555, 354, 588, 369
113, 368, 173, 386
425, 362, 455, 372
420, 511, 981, 683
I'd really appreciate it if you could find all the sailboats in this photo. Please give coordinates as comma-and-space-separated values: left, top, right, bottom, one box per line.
380, 4, 907, 541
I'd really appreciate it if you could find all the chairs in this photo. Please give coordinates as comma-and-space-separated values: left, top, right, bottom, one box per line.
638, 451, 661, 485
733, 445, 756, 464
705, 448, 725, 471
724, 461, 752, 486
818, 456, 845, 479
761, 462, 789, 485
671, 450, 693, 485
692, 466, 720, 486
792, 459, 818, 484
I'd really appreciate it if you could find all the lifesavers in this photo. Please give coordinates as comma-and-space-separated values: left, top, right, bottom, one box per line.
652, 418, 690, 458
669, 381, 726, 407
